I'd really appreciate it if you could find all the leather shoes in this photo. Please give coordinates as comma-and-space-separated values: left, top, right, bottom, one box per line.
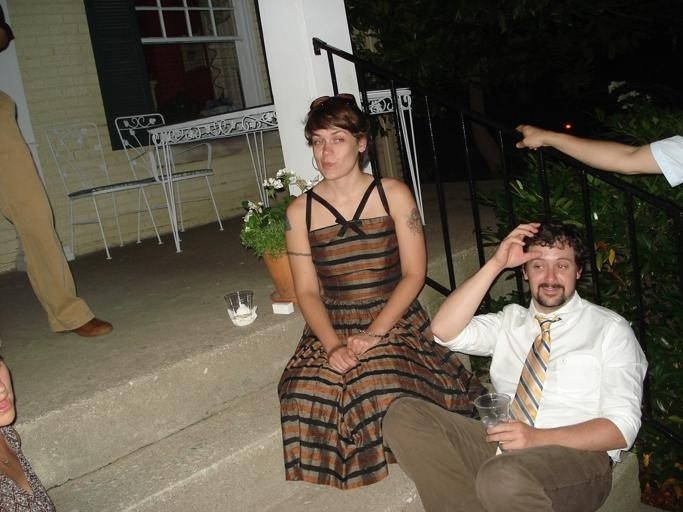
59, 318, 112, 337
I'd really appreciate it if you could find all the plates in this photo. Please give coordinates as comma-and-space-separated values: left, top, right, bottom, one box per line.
227, 304, 258, 327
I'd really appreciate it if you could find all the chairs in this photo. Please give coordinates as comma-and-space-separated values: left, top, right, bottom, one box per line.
44, 113, 225, 261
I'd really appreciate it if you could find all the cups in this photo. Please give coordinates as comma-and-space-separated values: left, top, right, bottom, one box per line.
224, 290, 254, 314
475, 393, 511, 433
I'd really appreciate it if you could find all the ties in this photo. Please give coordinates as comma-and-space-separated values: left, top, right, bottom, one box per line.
508, 315, 561, 428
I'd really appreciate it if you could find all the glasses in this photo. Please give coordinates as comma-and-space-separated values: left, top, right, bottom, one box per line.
310, 94, 357, 109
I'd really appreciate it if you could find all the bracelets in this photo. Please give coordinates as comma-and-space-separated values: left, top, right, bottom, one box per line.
360, 329, 384, 339
327, 344, 346, 358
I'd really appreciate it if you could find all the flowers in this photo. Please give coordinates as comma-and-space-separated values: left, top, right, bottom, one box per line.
239, 167, 320, 262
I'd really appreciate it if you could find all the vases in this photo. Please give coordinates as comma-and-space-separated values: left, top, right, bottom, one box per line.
261, 248, 296, 297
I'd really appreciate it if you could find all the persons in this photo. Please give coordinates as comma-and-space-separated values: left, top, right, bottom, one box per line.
0, 356, 55, 512
381, 223, 650, 512
0, 8, 113, 337
275, 95, 488, 489
515, 123, 683, 188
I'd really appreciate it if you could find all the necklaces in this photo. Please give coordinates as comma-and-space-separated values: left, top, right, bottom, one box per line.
0, 441, 11, 468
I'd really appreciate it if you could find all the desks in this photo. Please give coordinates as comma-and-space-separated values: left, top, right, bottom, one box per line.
150, 87, 427, 254
